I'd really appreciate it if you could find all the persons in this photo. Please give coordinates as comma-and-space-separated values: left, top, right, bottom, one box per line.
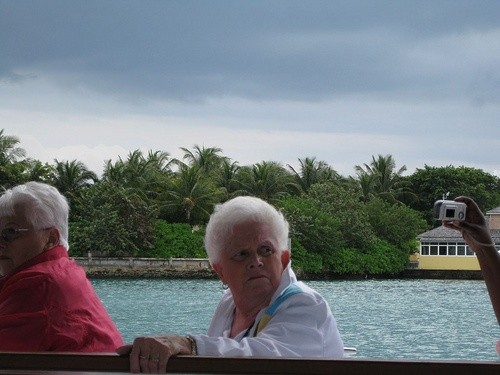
443, 196, 500, 351
115, 196, 345, 374
0, 182, 125, 353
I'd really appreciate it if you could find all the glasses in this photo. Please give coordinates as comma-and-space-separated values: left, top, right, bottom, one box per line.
0, 226, 51, 241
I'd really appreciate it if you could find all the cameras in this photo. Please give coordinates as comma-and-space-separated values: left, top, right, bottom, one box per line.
433, 200, 466, 220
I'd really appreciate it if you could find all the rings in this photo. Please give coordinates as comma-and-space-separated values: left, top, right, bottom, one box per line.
140, 355, 150, 360
150, 356, 160, 363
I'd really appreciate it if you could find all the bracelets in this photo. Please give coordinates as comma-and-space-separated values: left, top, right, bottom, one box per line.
186, 335, 197, 355
